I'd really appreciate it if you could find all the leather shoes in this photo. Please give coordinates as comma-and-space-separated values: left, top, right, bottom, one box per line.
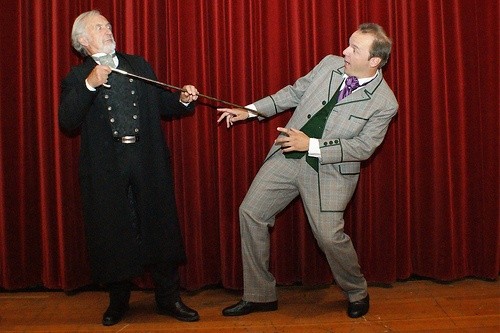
154, 295, 201, 322
222, 296, 279, 315
348, 292, 370, 317
101, 294, 130, 326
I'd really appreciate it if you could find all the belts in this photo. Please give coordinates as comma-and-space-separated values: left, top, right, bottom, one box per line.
114, 134, 139, 143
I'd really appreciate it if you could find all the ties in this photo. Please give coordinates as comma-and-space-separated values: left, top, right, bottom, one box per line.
338, 74, 359, 102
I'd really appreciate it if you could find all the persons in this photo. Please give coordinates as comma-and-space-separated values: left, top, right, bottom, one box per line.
58, 11, 201, 326
217, 22, 400, 317
